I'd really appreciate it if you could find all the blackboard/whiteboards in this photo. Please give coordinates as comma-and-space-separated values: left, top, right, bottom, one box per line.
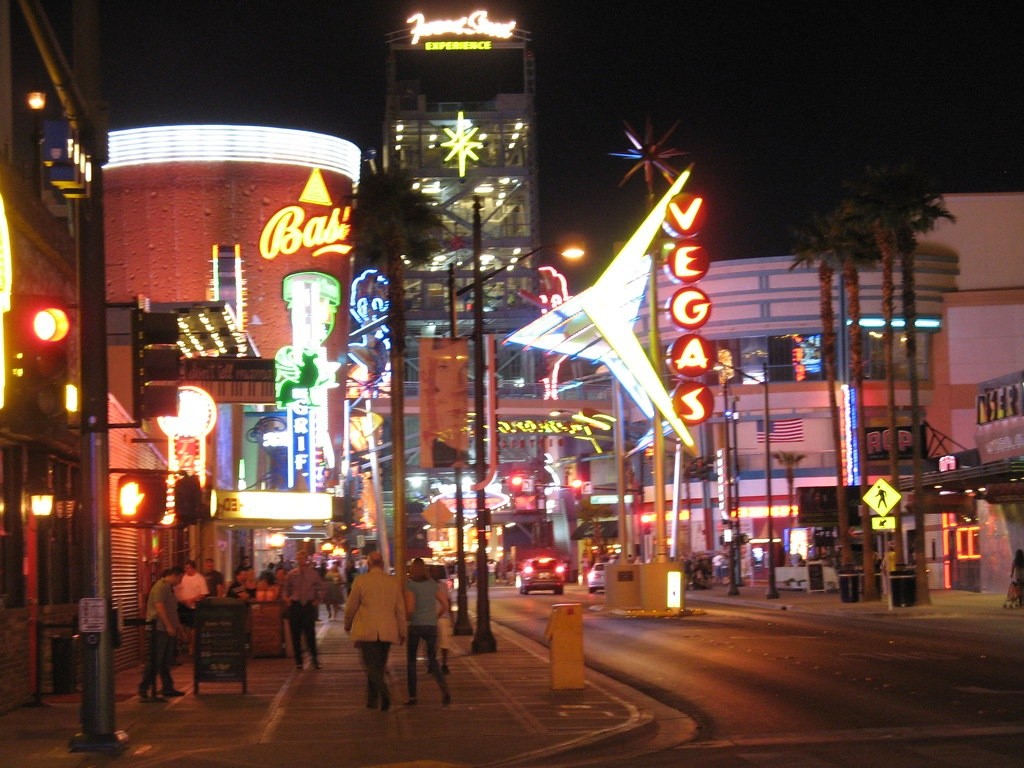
248, 602, 285, 658
194, 603, 250, 681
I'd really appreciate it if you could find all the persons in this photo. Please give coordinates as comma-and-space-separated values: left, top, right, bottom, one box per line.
506, 560, 515, 587
227, 566, 250, 599
246, 566, 258, 598
200, 558, 224, 598
487, 561, 497, 588
312, 560, 368, 617
678, 551, 730, 590
402, 558, 453, 706
255, 549, 327, 670
344, 550, 407, 711
172, 560, 209, 659
1009, 549, 1024, 606
425, 565, 451, 675
139, 565, 187, 702
612, 554, 644, 564
880, 545, 896, 572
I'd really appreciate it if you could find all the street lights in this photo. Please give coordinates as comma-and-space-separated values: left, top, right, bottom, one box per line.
28, 482, 56, 706
445, 235, 582, 638
713, 359, 780, 599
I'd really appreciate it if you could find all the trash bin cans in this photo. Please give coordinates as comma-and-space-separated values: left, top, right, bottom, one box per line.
838, 570, 860, 603
545, 603, 586, 688
888, 570, 919, 607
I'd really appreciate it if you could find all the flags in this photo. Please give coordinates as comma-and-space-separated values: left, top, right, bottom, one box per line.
757, 418, 805, 443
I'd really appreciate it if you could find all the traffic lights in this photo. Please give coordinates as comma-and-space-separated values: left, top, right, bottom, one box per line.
700, 459, 714, 480
180, 480, 218, 522
728, 509, 738, 520
571, 481, 583, 500
508, 476, 522, 496
115, 474, 165, 523
127, 305, 182, 426
12, 299, 69, 432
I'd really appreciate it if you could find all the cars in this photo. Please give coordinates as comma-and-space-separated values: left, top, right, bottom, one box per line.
514, 557, 565, 595
588, 564, 607, 592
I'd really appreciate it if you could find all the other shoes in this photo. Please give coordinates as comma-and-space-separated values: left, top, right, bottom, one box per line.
140, 684, 148, 698
311, 659, 322, 670
442, 692, 450, 706
296, 659, 303, 669
163, 687, 185, 697
381, 696, 389, 710
403, 697, 416, 704
441, 664, 449, 675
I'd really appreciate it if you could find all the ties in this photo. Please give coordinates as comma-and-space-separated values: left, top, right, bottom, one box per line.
300, 572, 308, 607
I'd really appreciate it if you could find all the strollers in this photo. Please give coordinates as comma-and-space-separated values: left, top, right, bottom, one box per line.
1002, 577, 1020, 609
